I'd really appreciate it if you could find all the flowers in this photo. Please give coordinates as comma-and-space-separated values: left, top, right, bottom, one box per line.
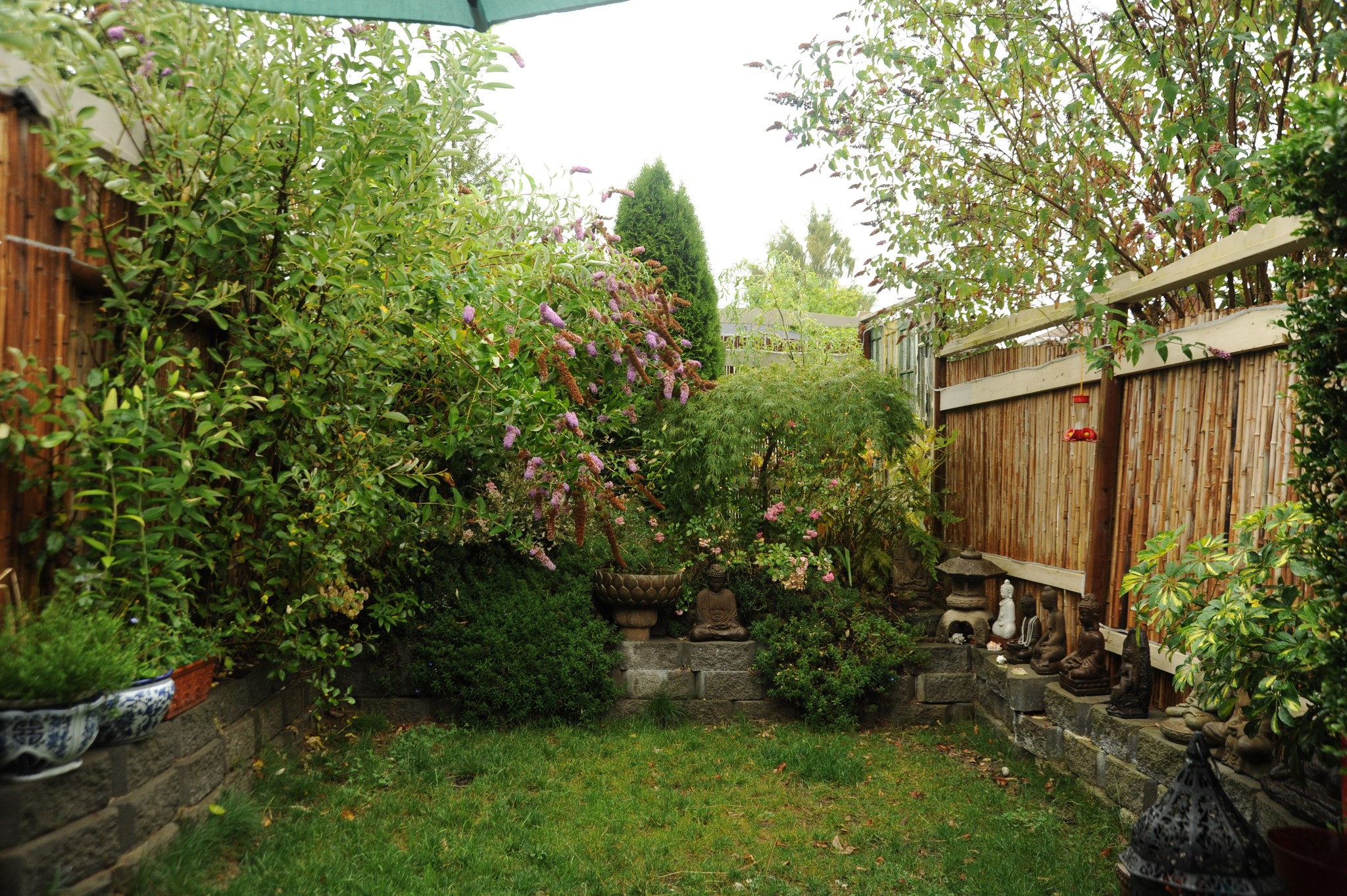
597, 492, 687, 574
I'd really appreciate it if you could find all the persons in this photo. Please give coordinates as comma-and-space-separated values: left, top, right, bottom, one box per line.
1032, 586, 1067, 667
1165, 654, 1277, 761
1109, 648, 1134, 700
690, 564, 748, 641
992, 579, 1016, 639
1061, 593, 1106, 680
1006, 591, 1043, 660
898, 549, 926, 586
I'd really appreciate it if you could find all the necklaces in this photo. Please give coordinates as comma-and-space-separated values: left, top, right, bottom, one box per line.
1018, 615, 1038, 646
999, 599, 1013, 606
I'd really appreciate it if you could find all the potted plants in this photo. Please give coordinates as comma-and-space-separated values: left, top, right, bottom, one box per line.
1266, 816, 1347, 896
1, 321, 264, 783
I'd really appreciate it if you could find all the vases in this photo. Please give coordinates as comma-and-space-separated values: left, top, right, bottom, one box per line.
595, 569, 685, 644
